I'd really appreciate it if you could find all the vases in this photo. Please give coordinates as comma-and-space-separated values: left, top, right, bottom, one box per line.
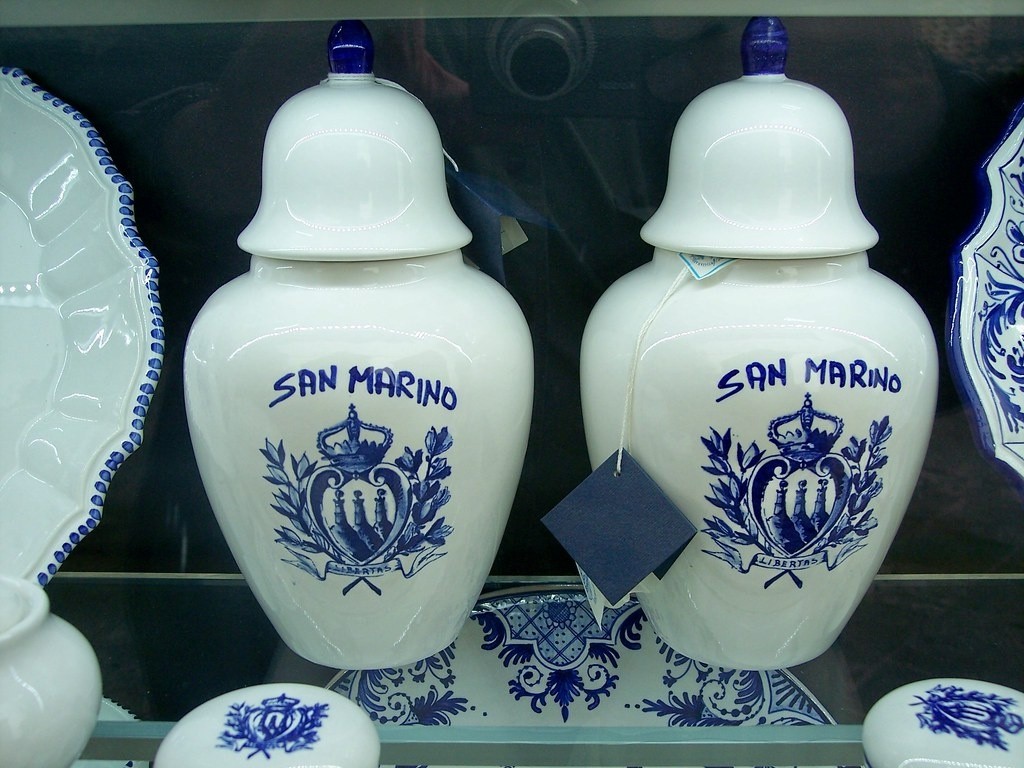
580, 20, 941, 673
179, 23, 537, 673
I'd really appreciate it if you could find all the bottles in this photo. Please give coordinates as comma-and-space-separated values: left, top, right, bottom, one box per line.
581, 17, 940, 672
184, 21, 533, 668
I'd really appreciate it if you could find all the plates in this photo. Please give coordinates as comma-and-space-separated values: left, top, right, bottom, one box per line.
0, 64, 165, 591
327, 584, 867, 767
947, 102, 1024, 501
